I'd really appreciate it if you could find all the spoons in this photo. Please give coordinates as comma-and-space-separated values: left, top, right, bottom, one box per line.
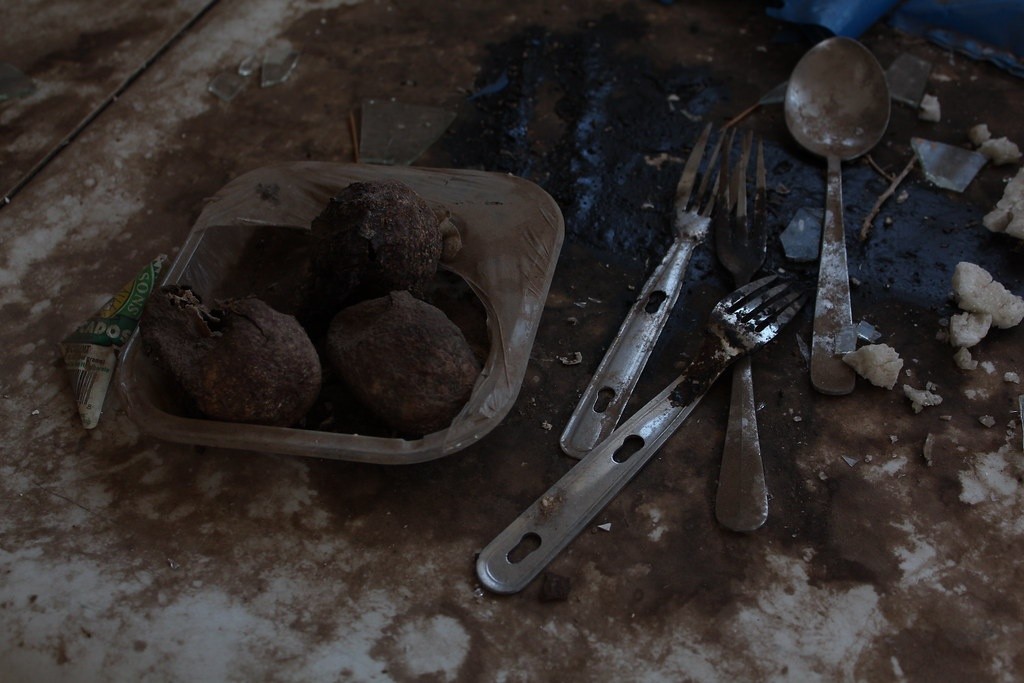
786, 34, 892, 393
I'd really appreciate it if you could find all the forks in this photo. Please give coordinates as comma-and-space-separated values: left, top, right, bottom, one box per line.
477, 274, 810, 594
711, 124, 770, 533
560, 121, 741, 460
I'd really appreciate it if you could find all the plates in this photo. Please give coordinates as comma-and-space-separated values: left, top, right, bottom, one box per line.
115, 161, 566, 464
